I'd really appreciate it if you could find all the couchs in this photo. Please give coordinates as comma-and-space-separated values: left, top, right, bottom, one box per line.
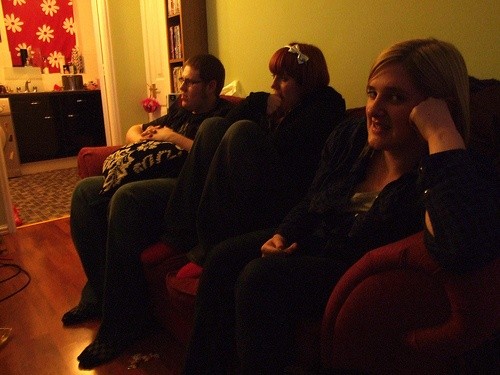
78, 94, 500, 375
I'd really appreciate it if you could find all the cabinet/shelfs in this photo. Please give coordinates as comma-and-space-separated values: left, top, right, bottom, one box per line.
10, 90, 106, 163
164, 0, 208, 94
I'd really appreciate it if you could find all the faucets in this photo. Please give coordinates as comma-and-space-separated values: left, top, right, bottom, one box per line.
24, 81, 30, 91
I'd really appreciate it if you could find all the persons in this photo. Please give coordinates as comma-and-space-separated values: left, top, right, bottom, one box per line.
138, 42, 346, 279
60, 54, 235, 367
183, 39, 500, 375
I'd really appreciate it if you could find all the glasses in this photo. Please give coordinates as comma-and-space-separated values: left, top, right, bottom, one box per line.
178, 78, 207, 87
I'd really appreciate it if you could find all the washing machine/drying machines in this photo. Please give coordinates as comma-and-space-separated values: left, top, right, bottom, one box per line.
0, 97, 21, 179
